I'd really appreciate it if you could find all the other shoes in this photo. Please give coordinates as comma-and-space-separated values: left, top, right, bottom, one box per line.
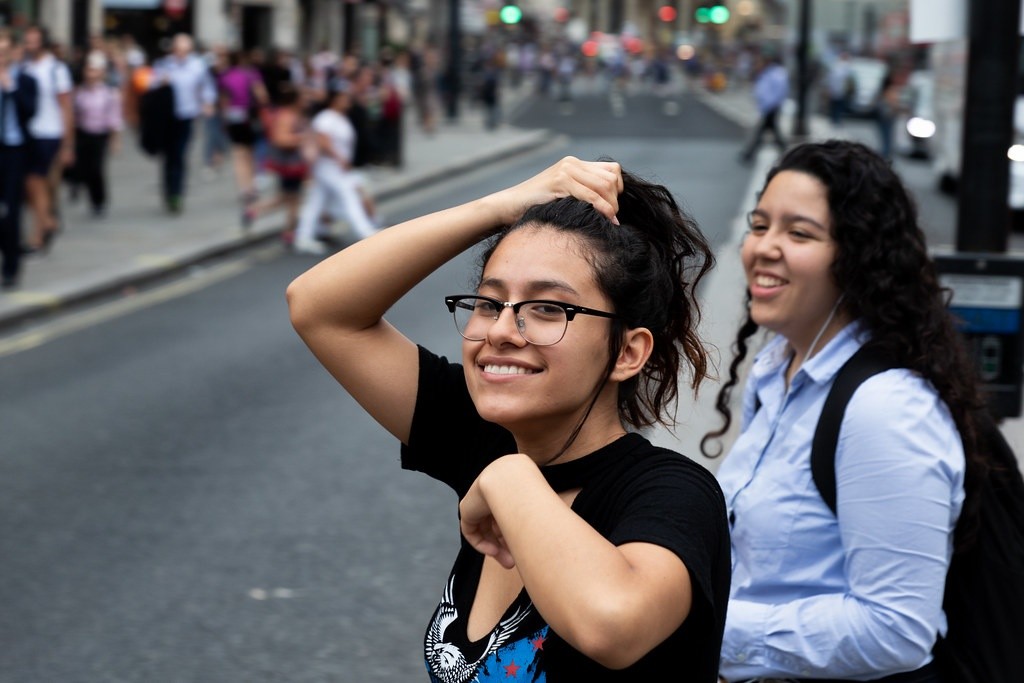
297, 241, 328, 256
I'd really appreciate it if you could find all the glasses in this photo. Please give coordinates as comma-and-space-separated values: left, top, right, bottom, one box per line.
444, 294, 619, 345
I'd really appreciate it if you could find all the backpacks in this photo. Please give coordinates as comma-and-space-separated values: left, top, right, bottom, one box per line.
754, 340, 1024, 683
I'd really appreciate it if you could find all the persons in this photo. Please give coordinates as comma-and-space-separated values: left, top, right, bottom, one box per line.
0, 22, 903, 284
286, 154, 732, 683
711, 137, 981, 683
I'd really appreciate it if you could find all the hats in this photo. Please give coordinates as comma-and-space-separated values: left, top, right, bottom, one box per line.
87, 51, 109, 74
